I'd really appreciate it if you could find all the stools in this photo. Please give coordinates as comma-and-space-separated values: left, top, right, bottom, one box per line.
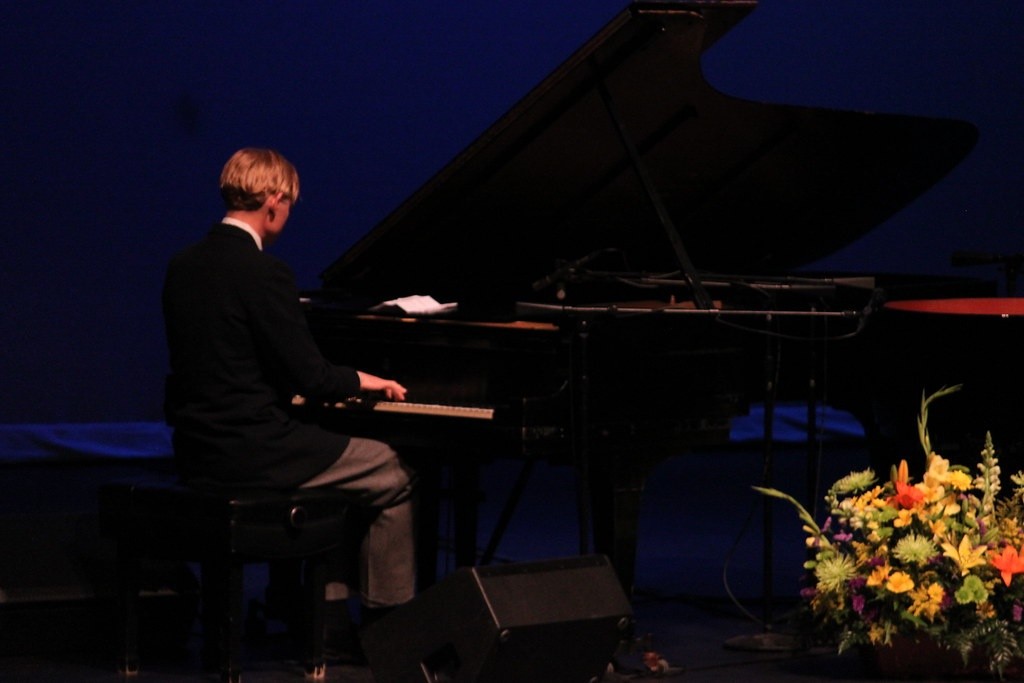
101, 480, 371, 683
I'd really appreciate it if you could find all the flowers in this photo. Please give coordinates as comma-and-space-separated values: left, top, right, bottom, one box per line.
751, 385, 1024, 683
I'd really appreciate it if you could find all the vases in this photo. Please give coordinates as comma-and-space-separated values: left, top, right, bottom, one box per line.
876, 631, 1024, 682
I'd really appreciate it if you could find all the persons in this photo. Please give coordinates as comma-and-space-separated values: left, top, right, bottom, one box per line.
161, 149, 414, 663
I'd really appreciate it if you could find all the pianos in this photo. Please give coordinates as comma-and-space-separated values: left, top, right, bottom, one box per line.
289, 0, 980, 678
